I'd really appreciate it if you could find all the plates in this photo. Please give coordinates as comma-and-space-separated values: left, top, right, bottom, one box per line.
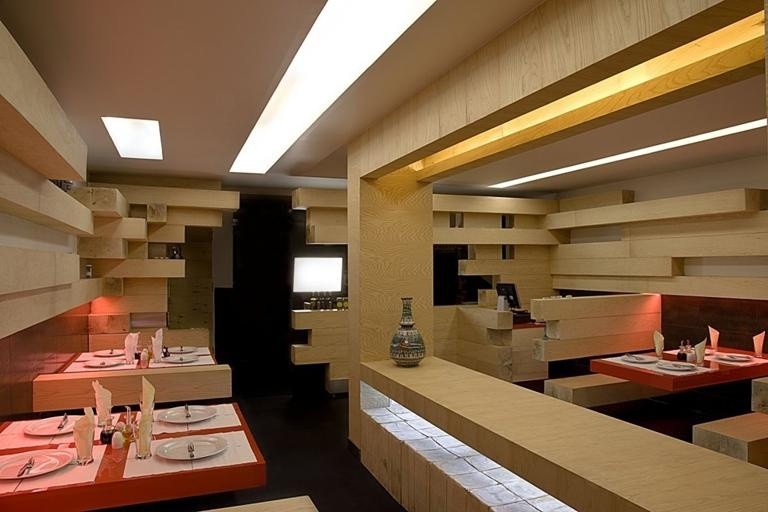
83, 359, 122, 367
720, 354, 752, 361
157, 404, 218, 423
655, 362, 697, 371
0, 448, 76, 480
703, 349, 716, 354
622, 356, 658, 363
157, 434, 229, 461
92, 349, 126, 357
162, 346, 197, 354
160, 355, 199, 363
22, 415, 84, 437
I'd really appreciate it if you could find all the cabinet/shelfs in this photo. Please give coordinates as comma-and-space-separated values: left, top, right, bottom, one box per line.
51, 178, 186, 282
347, 356, 768, 512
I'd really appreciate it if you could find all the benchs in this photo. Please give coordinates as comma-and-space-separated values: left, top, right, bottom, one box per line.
174, 495, 319, 512
691, 411, 768, 470
543, 373, 672, 407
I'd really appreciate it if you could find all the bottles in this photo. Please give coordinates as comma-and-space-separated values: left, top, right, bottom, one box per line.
84, 264, 94, 278
99, 416, 116, 444
111, 427, 123, 450
309, 296, 348, 310
140, 348, 148, 369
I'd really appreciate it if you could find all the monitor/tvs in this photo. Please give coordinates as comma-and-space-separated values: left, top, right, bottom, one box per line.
289, 252, 348, 298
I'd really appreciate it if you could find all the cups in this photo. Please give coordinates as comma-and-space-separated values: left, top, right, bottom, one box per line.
695, 346, 704, 367
654, 339, 664, 358
125, 347, 135, 364
94, 392, 112, 427
138, 391, 155, 422
753, 338, 764, 357
133, 419, 153, 459
152, 343, 162, 362
710, 334, 718, 349
70, 424, 94, 465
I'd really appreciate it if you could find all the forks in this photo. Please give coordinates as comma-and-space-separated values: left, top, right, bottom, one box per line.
187, 441, 196, 459
183, 403, 191, 418
58, 412, 68, 428
15, 456, 35, 477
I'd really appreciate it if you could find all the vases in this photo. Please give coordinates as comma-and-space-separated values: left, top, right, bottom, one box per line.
387, 296, 427, 367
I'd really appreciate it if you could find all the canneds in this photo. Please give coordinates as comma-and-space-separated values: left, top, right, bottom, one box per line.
85, 265, 93, 279
